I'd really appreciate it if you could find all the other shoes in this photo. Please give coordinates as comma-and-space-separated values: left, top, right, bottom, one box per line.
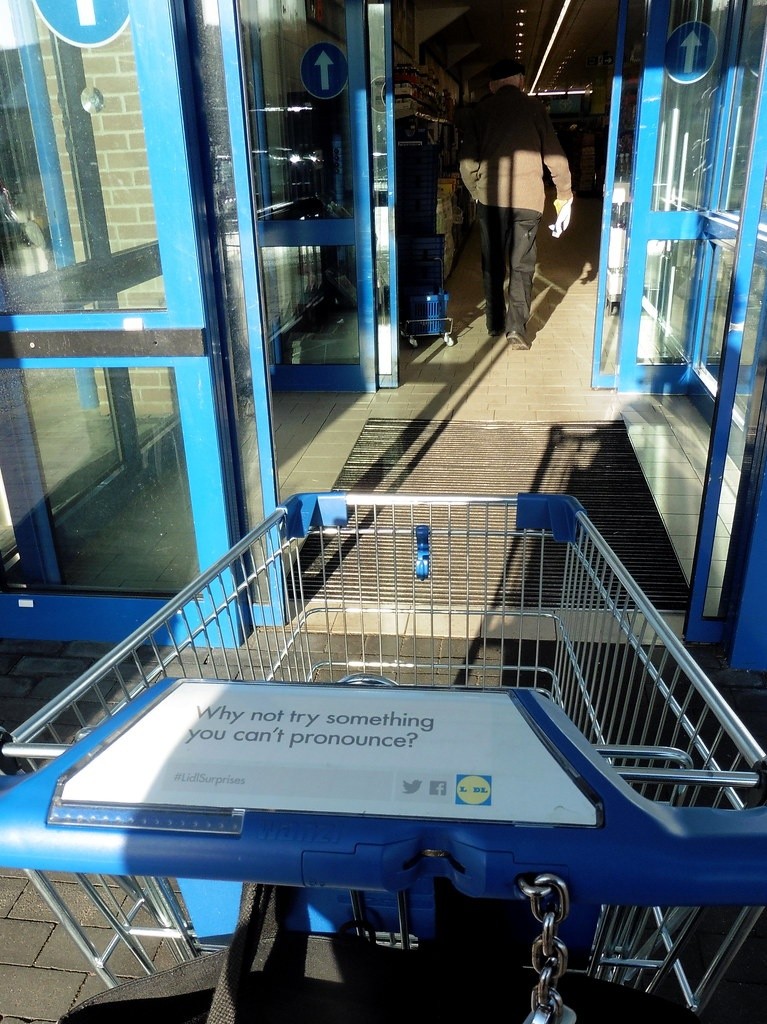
507, 331, 531, 350
488, 328, 505, 336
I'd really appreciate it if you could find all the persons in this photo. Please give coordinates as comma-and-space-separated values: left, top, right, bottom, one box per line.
460, 60, 575, 346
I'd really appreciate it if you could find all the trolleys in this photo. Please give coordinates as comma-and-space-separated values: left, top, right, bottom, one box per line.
0, 492, 767, 1015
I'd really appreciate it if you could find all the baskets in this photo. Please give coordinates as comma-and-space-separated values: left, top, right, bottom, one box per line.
394, 137, 449, 333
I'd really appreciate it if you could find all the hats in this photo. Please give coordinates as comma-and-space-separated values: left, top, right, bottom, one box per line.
488, 55, 522, 81
